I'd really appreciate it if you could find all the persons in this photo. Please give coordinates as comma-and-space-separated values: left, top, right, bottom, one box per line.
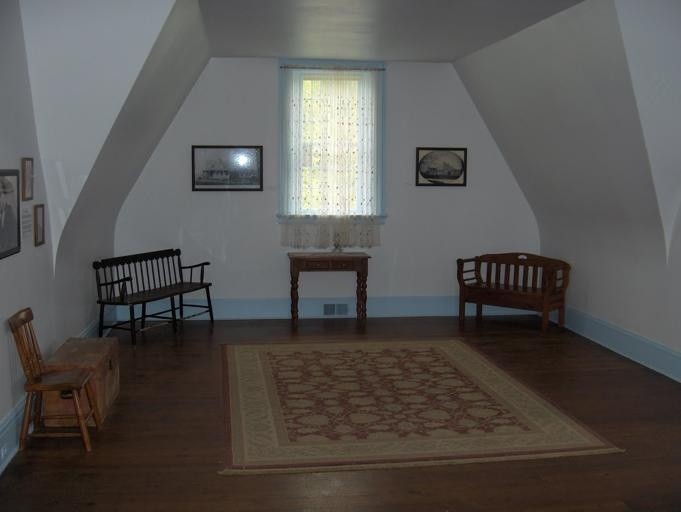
0, 177, 15, 248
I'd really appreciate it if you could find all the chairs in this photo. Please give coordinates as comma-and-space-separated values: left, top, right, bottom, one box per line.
9, 307, 103, 453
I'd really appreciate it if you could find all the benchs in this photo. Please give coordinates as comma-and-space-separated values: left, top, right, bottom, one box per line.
92, 249, 214, 344
456, 252, 571, 333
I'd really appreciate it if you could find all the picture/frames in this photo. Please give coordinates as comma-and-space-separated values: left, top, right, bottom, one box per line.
192, 144, 265, 191
33, 204, 46, 247
21, 157, 35, 201
0, 169, 22, 260
415, 147, 467, 187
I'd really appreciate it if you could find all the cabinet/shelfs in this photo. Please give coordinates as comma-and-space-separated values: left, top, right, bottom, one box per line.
41, 335, 121, 427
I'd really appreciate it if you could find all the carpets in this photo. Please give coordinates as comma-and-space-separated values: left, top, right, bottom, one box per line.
221, 336, 631, 476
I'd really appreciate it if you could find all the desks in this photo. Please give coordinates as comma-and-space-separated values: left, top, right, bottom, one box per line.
288, 251, 373, 325
